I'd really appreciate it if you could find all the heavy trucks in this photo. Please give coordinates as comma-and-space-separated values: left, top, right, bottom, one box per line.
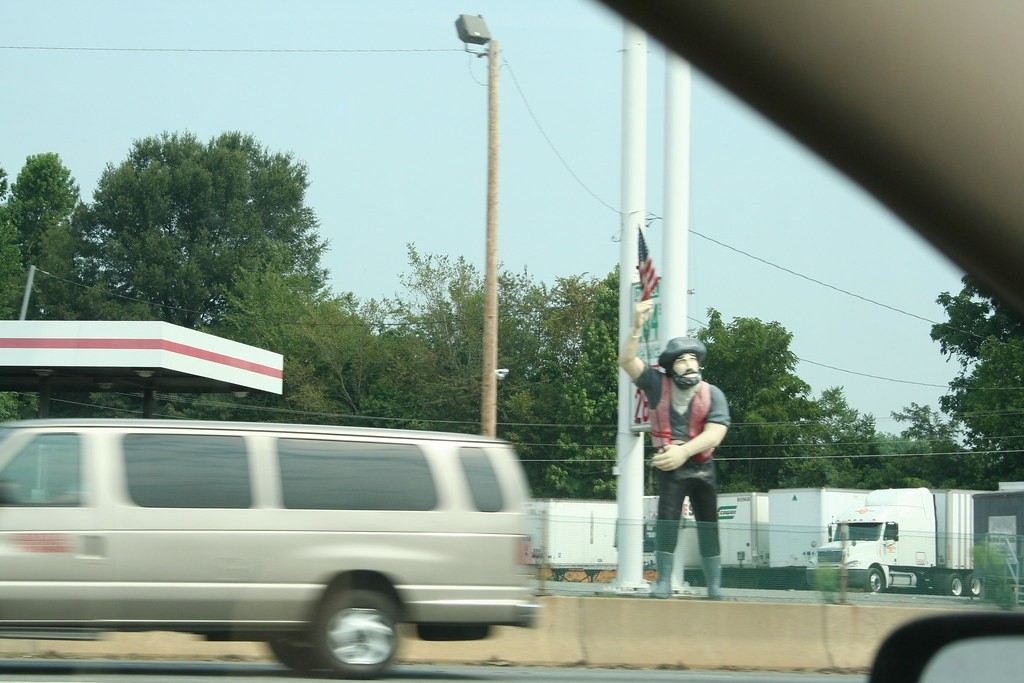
806, 486, 1017, 597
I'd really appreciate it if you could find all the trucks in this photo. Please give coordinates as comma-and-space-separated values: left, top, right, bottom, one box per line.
526, 487, 872, 588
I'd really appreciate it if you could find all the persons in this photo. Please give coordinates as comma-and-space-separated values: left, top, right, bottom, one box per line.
617, 299, 731, 602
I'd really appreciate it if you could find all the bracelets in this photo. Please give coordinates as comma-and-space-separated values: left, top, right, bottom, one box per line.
629, 327, 642, 339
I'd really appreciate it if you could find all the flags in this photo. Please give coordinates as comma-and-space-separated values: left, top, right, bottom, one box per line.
638, 225, 662, 302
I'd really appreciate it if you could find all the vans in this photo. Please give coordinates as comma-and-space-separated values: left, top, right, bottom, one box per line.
0, 419, 543, 681
